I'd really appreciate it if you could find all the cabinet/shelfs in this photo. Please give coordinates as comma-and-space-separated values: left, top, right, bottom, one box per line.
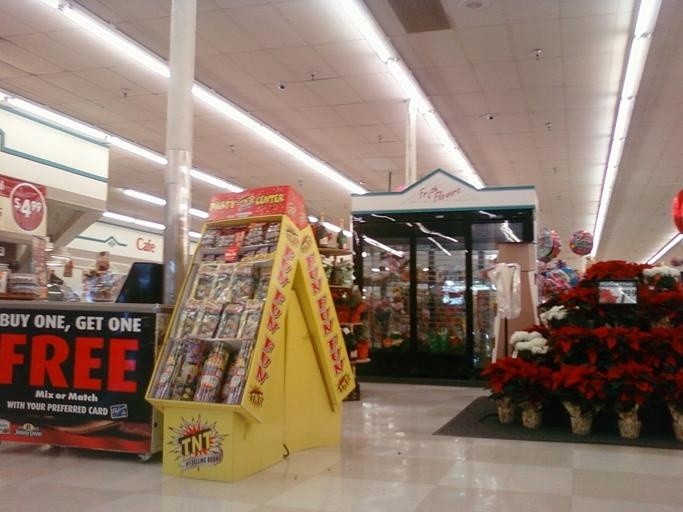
147, 215, 371, 421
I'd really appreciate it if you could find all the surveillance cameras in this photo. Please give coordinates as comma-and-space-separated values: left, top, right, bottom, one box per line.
488, 116, 494, 120
279, 84, 287, 90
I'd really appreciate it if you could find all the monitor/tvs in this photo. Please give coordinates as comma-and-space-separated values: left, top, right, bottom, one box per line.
596, 278, 639, 306
115, 262, 163, 303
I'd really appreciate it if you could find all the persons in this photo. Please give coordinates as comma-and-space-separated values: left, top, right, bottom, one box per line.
80, 250, 122, 303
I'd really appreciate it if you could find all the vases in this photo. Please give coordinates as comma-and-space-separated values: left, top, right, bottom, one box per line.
497, 398, 682, 440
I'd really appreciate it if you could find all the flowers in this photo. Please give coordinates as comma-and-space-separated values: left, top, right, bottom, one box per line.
475, 261, 683, 415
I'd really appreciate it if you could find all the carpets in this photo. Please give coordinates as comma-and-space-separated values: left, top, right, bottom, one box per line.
432, 395, 682, 450
